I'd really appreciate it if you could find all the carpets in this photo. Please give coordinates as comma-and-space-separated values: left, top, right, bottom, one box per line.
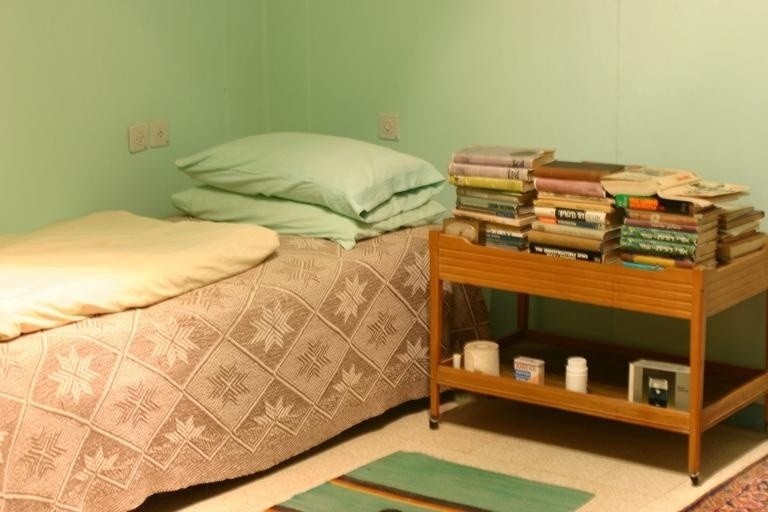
263, 448, 597, 511
682, 455, 768, 512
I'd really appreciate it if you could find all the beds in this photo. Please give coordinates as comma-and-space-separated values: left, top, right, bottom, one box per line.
0, 129, 484, 510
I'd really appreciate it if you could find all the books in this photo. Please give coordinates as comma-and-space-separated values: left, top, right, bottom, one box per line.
448, 145, 767, 274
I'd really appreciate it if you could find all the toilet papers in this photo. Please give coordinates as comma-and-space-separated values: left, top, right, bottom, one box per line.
463, 341, 499, 375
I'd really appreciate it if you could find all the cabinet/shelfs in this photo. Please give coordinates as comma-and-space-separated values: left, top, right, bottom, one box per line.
429, 228, 768, 489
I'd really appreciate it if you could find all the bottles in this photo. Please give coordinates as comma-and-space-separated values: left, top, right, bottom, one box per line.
565, 357, 589, 393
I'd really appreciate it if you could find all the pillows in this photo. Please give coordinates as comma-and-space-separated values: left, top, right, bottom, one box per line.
172, 132, 447, 223
172, 181, 447, 247
